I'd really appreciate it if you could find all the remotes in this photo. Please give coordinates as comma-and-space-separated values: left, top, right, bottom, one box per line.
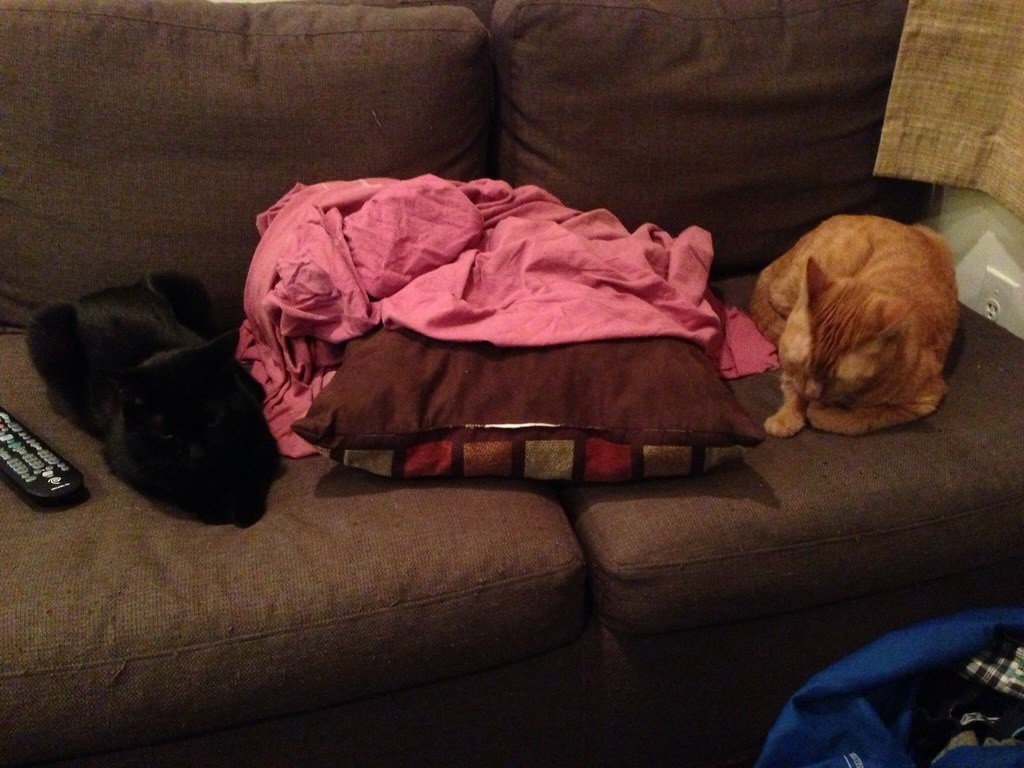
0, 405, 85, 504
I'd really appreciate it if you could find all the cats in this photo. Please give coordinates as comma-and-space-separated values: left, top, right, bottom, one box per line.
751, 214, 962, 438
26, 267, 281, 528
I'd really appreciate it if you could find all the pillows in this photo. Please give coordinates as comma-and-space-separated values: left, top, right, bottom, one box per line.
294, 323, 768, 484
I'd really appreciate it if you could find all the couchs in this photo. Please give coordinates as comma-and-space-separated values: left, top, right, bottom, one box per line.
0, 2, 1024, 768
